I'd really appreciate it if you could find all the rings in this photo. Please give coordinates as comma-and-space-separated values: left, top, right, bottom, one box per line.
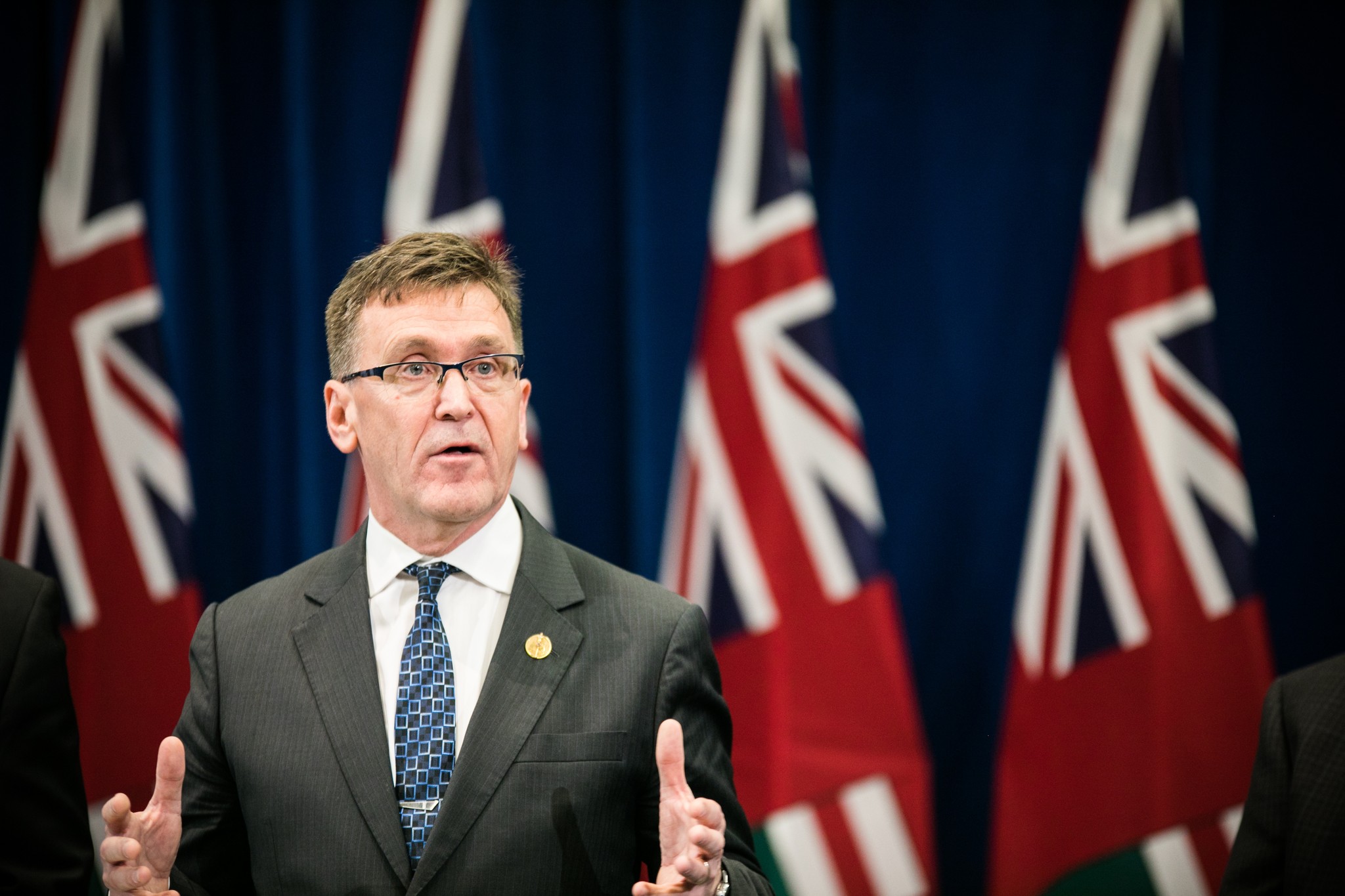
690, 861, 710, 884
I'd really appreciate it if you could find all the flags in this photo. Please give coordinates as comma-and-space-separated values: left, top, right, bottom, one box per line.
0, 0, 205, 876
990, 0, 1277, 896
658, 1, 935, 896
331, 0, 551, 549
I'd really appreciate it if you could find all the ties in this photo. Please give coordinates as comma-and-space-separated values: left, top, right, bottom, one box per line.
395, 562, 463, 877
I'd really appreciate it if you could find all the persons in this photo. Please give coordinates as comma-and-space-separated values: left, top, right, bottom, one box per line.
98, 234, 777, 896
1218, 656, 1345, 895
0, 559, 105, 896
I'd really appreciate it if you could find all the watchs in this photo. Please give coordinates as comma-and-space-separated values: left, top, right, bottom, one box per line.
715, 868, 730, 896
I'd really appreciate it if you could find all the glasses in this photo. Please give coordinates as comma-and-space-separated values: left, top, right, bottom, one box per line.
340, 353, 527, 401
